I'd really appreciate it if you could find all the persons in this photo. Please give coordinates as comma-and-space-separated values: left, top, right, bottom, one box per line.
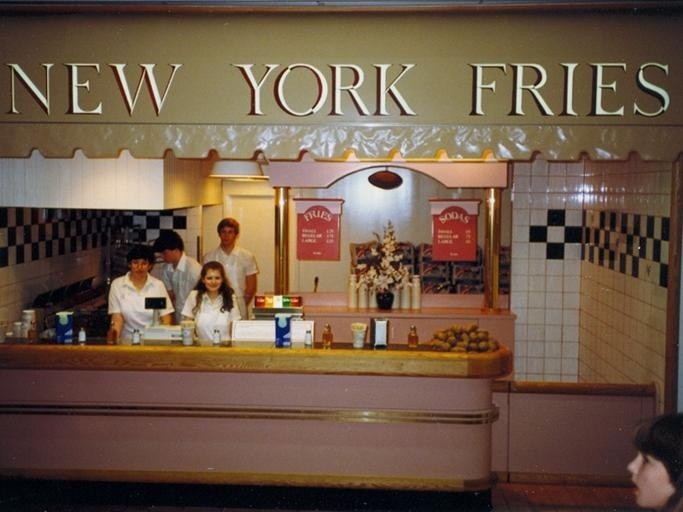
153, 230, 203, 324
108, 245, 175, 338
202, 217, 259, 320
627, 412, 683, 512
180, 261, 242, 341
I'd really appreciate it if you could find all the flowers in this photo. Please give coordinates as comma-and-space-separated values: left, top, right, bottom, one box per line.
348, 219, 420, 292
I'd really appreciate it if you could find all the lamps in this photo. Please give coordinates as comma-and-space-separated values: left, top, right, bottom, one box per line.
368, 166, 403, 190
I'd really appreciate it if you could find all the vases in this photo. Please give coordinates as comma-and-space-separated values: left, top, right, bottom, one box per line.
376, 291, 394, 308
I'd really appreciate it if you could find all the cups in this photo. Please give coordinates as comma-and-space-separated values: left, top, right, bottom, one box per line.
180, 322, 196, 346
348, 320, 368, 349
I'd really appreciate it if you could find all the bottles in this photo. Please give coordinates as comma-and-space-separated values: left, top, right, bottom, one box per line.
392, 289, 400, 308
56, 312, 74, 344
275, 315, 292, 346
367, 265, 378, 308
78, 326, 86, 342
401, 272, 410, 308
359, 272, 368, 308
409, 326, 418, 348
305, 330, 312, 349
412, 274, 421, 310
214, 330, 220, 344
133, 329, 140, 344
322, 323, 332, 348
347, 274, 358, 308
107, 322, 117, 344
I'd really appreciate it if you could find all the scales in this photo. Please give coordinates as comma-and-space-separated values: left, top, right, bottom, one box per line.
143, 297, 197, 346
